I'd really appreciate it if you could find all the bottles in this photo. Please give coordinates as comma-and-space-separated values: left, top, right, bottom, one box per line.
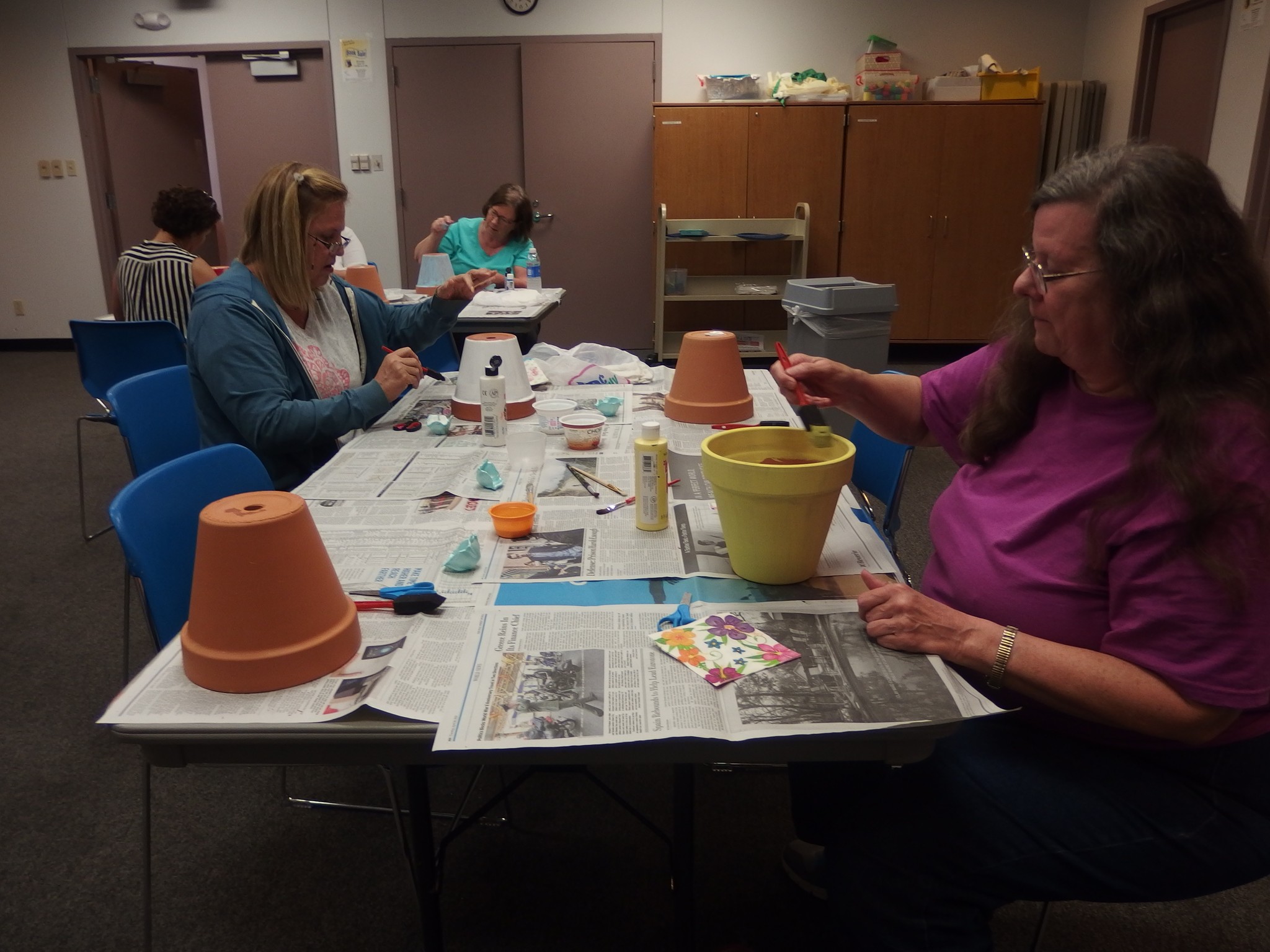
526, 248, 542, 295
506, 273, 514, 292
635, 423, 673, 531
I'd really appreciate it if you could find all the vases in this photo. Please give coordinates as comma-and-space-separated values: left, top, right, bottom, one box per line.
181, 489, 358, 696
663, 330, 753, 424
701, 426, 859, 584
343, 265, 389, 306
448, 333, 535, 421
415, 252, 455, 297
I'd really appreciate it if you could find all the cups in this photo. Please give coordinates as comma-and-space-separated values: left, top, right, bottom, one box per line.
664, 267, 689, 296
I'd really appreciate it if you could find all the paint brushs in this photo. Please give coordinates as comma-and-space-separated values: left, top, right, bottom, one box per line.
526, 484, 535, 522
381, 345, 446, 382
596, 477, 681, 515
566, 462, 600, 497
571, 465, 627, 497
774, 340, 827, 433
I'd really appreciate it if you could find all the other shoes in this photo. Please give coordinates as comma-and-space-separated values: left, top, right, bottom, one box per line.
781, 839, 830, 901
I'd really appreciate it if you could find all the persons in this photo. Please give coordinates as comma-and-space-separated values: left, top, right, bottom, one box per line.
414, 182, 538, 288
110, 182, 218, 340
186, 161, 497, 492
769, 141, 1269, 950
331, 225, 368, 268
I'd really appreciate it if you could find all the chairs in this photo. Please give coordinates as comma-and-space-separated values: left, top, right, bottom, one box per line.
99, 369, 213, 687
105, 442, 412, 952
1011, 856, 1270, 952
65, 319, 190, 545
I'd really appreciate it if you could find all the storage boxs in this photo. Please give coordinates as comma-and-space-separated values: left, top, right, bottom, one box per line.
853, 35, 918, 100
925, 73, 981, 101
978, 64, 1040, 99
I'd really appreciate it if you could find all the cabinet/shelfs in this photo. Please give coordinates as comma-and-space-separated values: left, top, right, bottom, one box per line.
644, 202, 812, 369
651, 100, 847, 331
846, 102, 1045, 343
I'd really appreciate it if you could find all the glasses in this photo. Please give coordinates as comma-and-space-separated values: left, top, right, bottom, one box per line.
488, 205, 517, 227
307, 232, 352, 257
1019, 244, 1109, 296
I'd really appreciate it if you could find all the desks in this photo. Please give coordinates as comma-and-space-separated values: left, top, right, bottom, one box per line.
364, 287, 570, 339
107, 339, 1005, 952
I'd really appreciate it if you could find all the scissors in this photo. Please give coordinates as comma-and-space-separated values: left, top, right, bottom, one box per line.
657, 590, 697, 634
391, 413, 422, 433
348, 581, 437, 601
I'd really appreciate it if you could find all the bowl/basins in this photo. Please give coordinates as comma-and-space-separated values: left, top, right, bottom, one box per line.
505, 431, 547, 470
489, 502, 538, 539
532, 398, 577, 435
558, 412, 607, 451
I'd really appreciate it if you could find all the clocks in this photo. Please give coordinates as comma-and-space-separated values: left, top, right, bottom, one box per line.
504, 0, 538, 15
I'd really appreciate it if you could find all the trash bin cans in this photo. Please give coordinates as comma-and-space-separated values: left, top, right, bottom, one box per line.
780, 275, 897, 447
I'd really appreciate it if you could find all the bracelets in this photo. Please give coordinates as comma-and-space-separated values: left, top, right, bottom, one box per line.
986, 624, 1019, 697
